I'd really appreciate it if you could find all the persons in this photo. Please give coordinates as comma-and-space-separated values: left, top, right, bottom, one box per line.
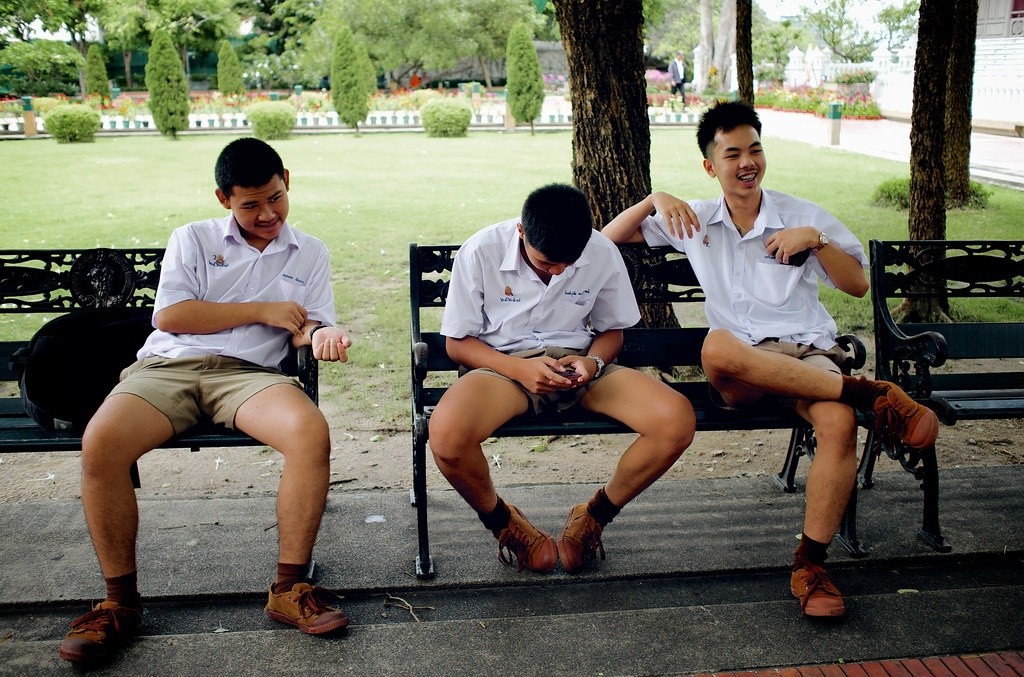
57, 136, 362, 667
427, 183, 697, 575
667, 49, 687, 102
599, 101, 940, 616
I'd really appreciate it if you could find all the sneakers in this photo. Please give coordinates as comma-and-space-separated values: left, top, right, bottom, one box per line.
873, 380, 939, 449
498, 504, 558, 574
266, 581, 350, 634
558, 502, 606, 573
790, 553, 845, 617
59, 600, 144, 662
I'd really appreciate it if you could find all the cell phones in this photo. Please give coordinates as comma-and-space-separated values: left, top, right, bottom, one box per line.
772, 248, 810, 268
554, 370, 582, 381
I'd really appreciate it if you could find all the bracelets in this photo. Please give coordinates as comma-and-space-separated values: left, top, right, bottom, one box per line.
310, 324, 327, 340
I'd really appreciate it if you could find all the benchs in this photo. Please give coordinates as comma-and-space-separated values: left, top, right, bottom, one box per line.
0, 247, 319, 582
858, 239, 1024, 553
409, 241, 866, 582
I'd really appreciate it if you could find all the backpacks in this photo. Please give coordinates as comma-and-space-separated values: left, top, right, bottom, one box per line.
12, 307, 154, 490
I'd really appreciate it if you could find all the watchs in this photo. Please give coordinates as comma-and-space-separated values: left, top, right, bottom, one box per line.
586, 354, 605, 380
810, 232, 830, 253
